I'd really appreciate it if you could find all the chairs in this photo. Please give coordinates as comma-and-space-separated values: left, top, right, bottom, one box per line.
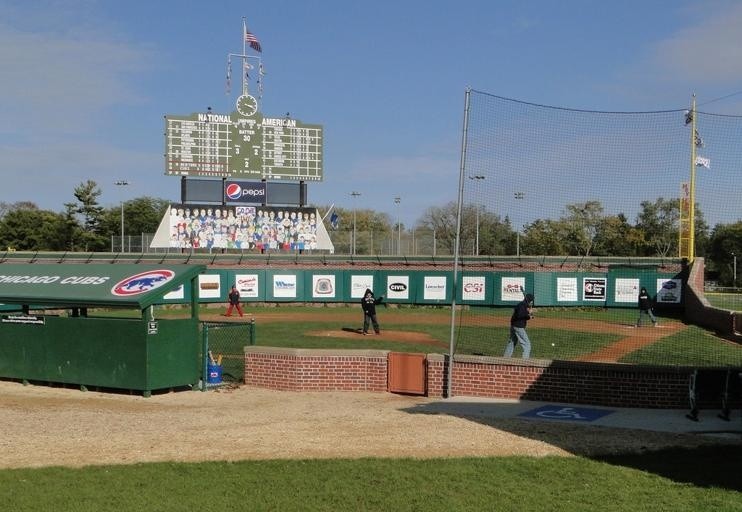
686, 368, 742, 421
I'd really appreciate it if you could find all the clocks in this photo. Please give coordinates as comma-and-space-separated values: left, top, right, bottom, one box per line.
236, 95, 258, 117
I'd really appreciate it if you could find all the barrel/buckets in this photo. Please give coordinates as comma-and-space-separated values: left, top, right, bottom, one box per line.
208, 364, 224, 383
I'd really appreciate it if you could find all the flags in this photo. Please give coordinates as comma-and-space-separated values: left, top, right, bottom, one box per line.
245, 30, 261, 53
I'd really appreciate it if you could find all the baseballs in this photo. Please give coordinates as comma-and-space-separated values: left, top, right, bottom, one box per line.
552, 344, 554, 345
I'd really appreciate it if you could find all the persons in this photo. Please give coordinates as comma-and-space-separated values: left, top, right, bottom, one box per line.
223, 285, 244, 318
361, 289, 383, 336
503, 294, 535, 359
636, 287, 655, 328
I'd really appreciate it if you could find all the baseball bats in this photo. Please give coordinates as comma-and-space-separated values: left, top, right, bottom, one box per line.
520, 286, 534, 319
